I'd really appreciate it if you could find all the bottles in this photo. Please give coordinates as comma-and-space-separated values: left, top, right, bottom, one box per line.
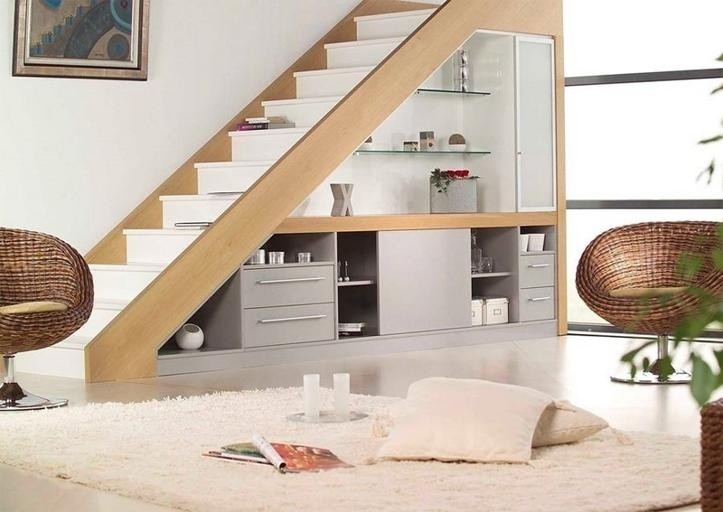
472, 232, 483, 276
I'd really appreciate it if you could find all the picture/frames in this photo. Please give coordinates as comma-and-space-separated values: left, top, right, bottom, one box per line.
11, 0, 151, 82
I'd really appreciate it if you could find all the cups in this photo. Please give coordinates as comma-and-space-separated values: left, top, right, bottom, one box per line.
481, 255, 494, 274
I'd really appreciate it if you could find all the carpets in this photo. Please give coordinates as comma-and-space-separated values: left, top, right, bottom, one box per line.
0, 381, 702, 512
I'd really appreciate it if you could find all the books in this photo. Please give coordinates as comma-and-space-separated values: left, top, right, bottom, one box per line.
173, 219, 212, 227
237, 117, 294, 131
205, 191, 245, 195
338, 320, 366, 337
202, 434, 358, 474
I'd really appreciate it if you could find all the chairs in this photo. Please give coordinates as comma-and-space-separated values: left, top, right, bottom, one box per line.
575, 220, 723, 385
0, 226, 95, 411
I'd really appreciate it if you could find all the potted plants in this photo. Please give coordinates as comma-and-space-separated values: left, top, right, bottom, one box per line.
621, 50, 723, 512
